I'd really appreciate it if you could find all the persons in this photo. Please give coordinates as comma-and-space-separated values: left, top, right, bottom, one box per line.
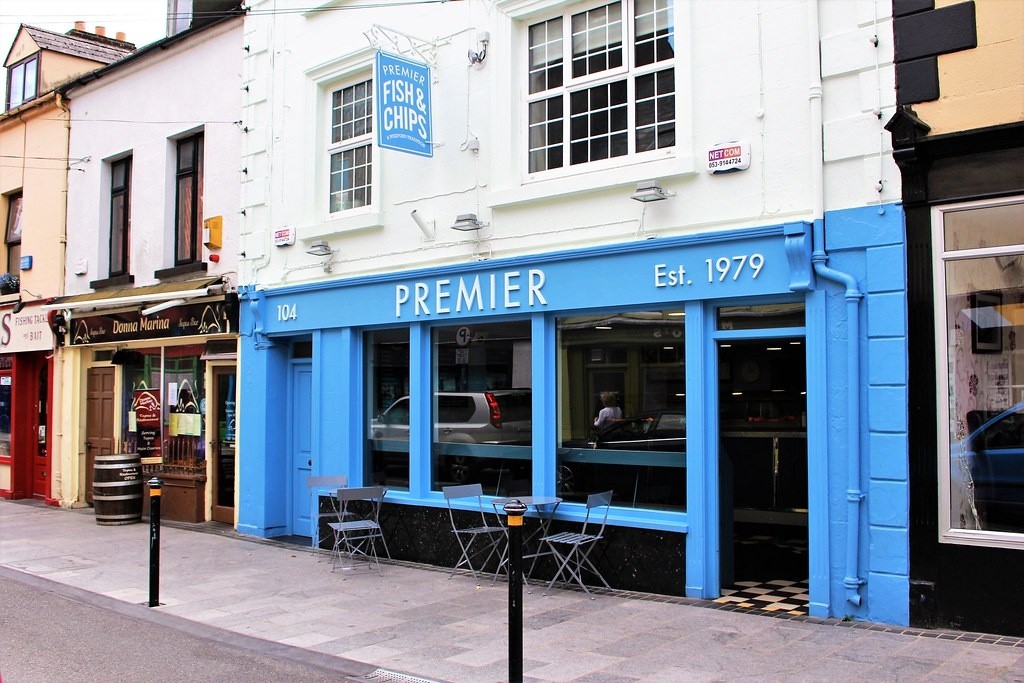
593, 391, 622, 430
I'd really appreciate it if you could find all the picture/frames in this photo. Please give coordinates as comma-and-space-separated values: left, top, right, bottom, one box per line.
970, 289, 1003, 355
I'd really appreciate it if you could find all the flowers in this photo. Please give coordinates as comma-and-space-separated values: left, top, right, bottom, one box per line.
0, 272, 20, 289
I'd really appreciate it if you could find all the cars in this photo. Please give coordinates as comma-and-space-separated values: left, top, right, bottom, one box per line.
555, 407, 686, 494
952, 402, 1024, 515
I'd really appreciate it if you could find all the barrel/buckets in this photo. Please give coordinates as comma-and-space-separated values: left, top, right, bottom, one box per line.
92, 454, 143, 526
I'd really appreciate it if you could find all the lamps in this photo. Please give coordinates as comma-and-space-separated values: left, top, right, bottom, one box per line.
306, 240, 341, 257
884, 108, 931, 138
450, 213, 490, 231
13, 288, 42, 314
629, 181, 677, 203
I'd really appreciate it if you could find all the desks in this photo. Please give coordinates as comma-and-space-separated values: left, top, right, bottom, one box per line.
328, 487, 397, 564
491, 496, 567, 594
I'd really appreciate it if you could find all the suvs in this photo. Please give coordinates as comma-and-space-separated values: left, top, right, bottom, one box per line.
369, 386, 535, 484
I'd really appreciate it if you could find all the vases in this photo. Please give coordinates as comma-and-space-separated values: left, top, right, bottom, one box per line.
0, 285, 19, 295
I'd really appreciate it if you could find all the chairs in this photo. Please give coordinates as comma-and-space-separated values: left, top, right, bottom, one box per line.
539, 489, 615, 600
306, 474, 357, 562
966, 410, 1024, 533
442, 483, 509, 590
327, 486, 385, 578
